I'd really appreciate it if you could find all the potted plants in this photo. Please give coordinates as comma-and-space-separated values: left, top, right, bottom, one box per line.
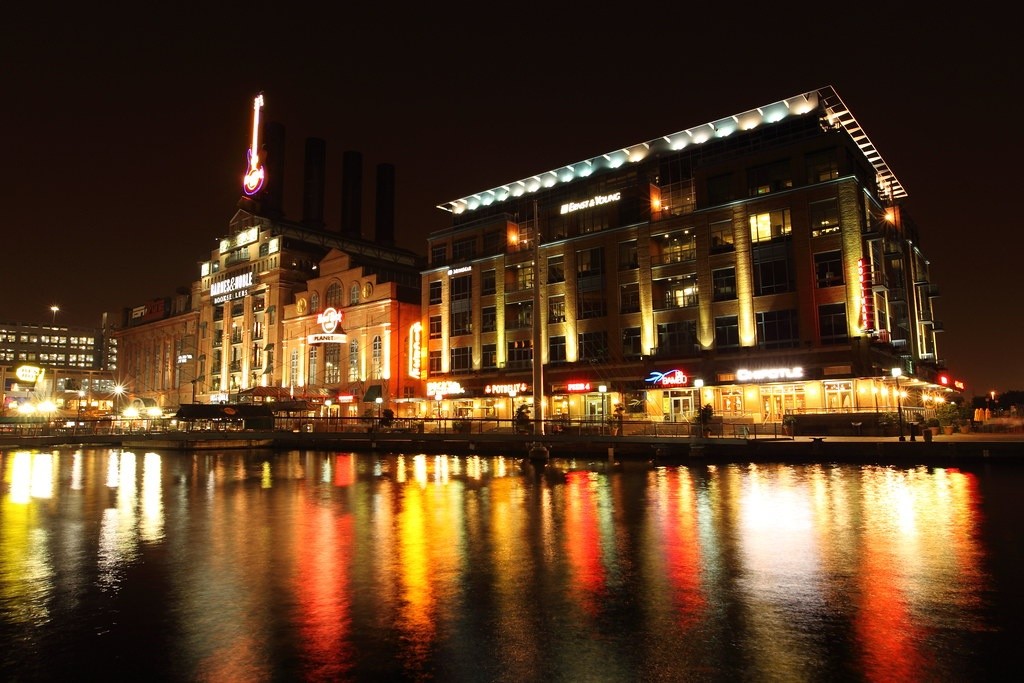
515, 405, 531, 434
913, 403, 971, 435
878, 412, 899, 437
696, 404, 715, 437
606, 417, 620, 436
782, 416, 796, 435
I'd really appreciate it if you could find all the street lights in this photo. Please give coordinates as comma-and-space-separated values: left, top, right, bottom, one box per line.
599, 386, 607, 435
892, 368, 906, 441
435, 395, 442, 433
113, 384, 122, 412
376, 398, 383, 432
38, 402, 56, 435
73, 389, 86, 433
509, 391, 516, 435
695, 379, 704, 438
